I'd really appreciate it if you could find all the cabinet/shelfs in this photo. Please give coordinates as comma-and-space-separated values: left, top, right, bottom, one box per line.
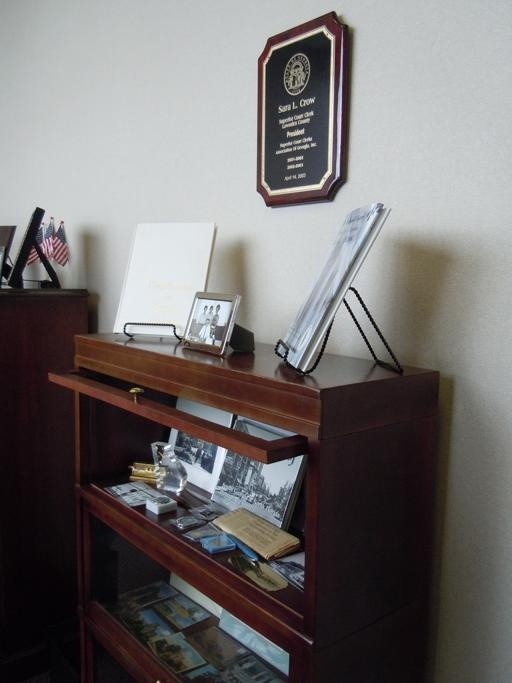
48, 331, 440, 683
0, 285, 120, 682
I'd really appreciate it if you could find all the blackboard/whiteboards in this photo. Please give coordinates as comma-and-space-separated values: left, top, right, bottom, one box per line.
256, 10, 349, 207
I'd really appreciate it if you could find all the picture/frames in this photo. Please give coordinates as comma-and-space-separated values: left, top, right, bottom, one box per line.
8, 206, 61, 288
1, 226, 23, 289
181, 292, 255, 357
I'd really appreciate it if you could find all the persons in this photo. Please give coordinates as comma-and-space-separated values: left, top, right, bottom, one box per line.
190, 304, 221, 344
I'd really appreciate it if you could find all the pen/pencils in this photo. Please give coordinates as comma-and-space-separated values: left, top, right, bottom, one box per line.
226, 533, 259, 561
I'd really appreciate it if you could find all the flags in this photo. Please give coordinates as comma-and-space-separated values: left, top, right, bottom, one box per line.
26, 224, 43, 266
43, 220, 56, 258
54, 222, 70, 266
